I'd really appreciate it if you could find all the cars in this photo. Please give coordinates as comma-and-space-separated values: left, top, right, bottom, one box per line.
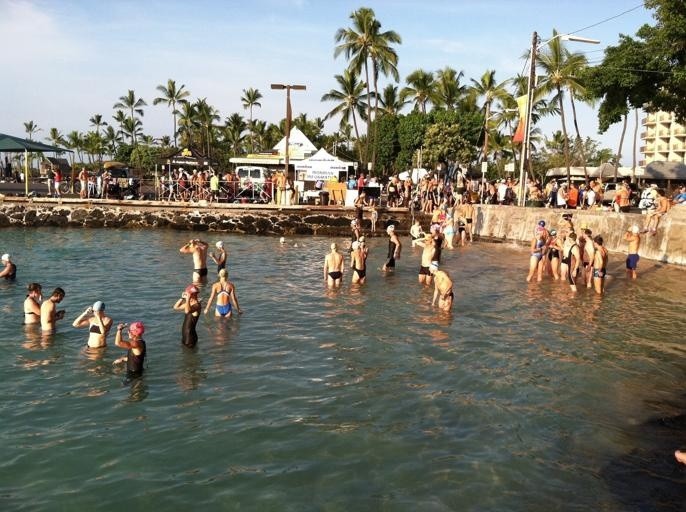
496, 179, 641, 208
88, 165, 143, 196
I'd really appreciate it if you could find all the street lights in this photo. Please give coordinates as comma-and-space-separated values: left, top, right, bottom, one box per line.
519, 31, 601, 207
481, 102, 539, 204
271, 84, 306, 204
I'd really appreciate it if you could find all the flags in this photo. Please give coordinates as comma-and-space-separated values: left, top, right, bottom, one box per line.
512, 95, 529, 143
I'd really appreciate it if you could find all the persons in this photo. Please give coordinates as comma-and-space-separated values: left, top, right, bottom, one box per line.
174, 284, 200, 348
408, 201, 476, 287
429, 260, 453, 312
203, 269, 244, 318
324, 154, 686, 235
381, 224, 402, 273
621, 225, 641, 279
323, 220, 369, 287
113, 320, 149, 372
1, 154, 295, 203
674, 449, 686, 464
71, 301, 113, 348
2, 253, 17, 278
23, 283, 66, 330
525, 214, 608, 296
179, 239, 227, 282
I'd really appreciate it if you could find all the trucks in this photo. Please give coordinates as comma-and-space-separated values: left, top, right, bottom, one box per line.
234, 165, 272, 204
166, 159, 215, 182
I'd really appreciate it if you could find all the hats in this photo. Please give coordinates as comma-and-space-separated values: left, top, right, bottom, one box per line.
580, 224, 588, 229
92, 301, 105, 311
536, 221, 557, 236
129, 322, 145, 338
352, 241, 361, 251
219, 269, 228, 278
359, 236, 365, 243
351, 220, 358, 225
387, 225, 395, 233
186, 285, 200, 298
331, 243, 337, 250
2, 254, 13, 261
430, 224, 440, 234
633, 226, 640, 234
425, 234, 432, 241
216, 241, 224, 248
430, 261, 439, 270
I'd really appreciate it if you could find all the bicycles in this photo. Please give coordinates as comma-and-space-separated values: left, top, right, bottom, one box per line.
58, 173, 82, 195
